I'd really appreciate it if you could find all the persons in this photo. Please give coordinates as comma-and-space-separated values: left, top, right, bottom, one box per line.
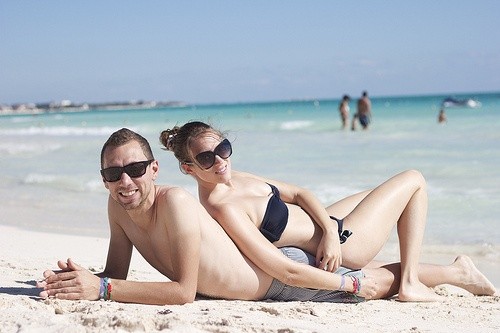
338, 95, 350, 128
438, 109, 447, 124
37, 128, 499, 305
352, 113, 360, 130
357, 91, 372, 130
157, 120, 448, 302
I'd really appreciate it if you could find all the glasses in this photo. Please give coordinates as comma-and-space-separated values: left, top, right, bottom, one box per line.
100, 159, 154, 182
181, 139, 232, 170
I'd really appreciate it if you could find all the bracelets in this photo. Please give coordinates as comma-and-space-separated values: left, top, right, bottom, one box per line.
98, 277, 115, 302
339, 275, 347, 291
351, 275, 362, 296
